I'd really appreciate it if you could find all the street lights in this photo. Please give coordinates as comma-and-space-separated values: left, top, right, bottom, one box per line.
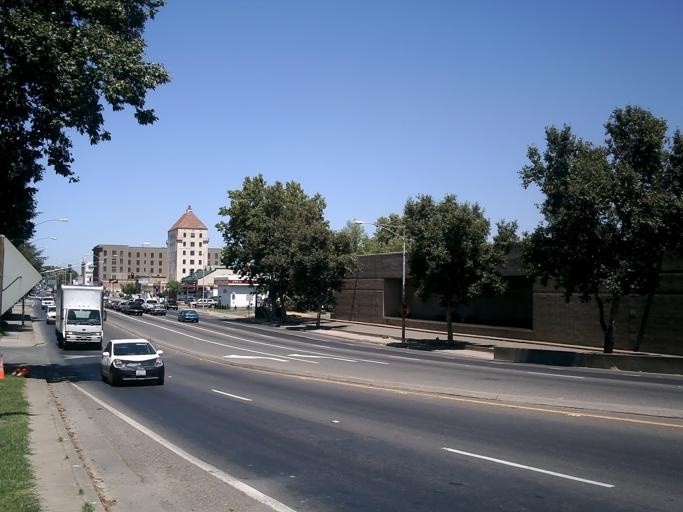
354, 218, 408, 349
25, 236, 58, 245
34, 218, 70, 227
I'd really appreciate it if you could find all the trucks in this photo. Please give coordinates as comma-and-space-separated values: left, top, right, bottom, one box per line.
55, 284, 104, 350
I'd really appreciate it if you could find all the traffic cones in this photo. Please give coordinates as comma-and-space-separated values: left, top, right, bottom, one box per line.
0, 354, 34, 380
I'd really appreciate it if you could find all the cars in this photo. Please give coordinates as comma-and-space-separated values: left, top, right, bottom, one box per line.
178, 310, 199, 323
99, 337, 165, 388
45, 306, 56, 325
103, 294, 167, 317
189, 299, 218, 309
40, 296, 55, 309
